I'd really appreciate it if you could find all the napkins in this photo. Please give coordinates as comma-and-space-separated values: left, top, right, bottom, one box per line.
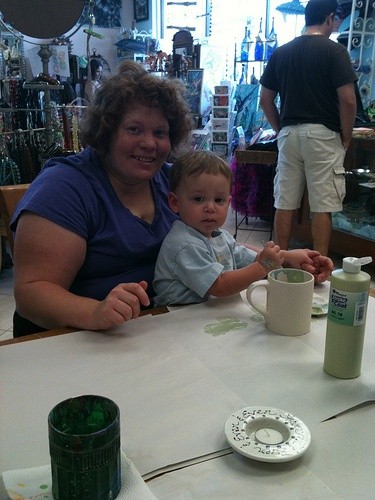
2, 449, 156, 500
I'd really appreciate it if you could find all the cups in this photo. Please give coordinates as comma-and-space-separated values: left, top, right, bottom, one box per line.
246, 269, 314, 336
48, 395, 121, 500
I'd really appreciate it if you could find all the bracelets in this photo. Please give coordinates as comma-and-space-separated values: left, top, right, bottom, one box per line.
253, 257, 277, 272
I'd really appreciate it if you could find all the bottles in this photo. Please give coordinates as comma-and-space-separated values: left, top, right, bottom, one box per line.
239, 64, 246, 84
254, 17, 263, 61
324, 257, 372, 378
241, 18, 254, 62
265, 17, 278, 60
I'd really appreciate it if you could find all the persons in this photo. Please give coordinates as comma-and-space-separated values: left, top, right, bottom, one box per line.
10, 60, 333, 338
258, 0, 359, 257
84, 59, 104, 105
151, 150, 322, 309
0, 38, 20, 60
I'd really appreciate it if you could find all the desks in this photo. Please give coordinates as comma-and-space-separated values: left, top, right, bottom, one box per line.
0, 274, 375, 500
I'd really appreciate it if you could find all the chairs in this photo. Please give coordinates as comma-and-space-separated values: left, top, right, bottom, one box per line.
0, 184, 35, 263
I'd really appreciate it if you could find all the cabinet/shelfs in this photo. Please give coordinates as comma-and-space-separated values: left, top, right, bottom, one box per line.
330, 135, 375, 267
